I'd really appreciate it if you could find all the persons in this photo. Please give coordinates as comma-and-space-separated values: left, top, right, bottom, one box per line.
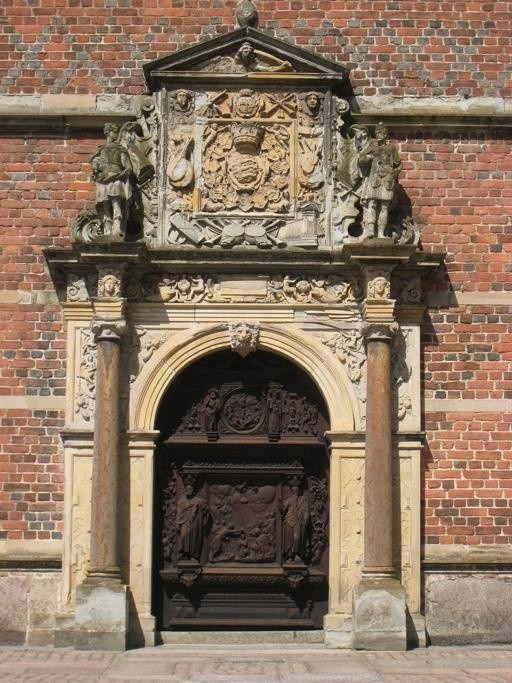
282, 273, 326, 302
236, 89, 257, 118
176, 88, 189, 107
208, 512, 275, 561
357, 121, 403, 241
211, 42, 293, 74
304, 91, 319, 110
175, 485, 200, 561
281, 486, 309, 564
264, 123, 290, 152
89, 122, 132, 238
200, 123, 229, 152
101, 274, 120, 297
267, 391, 282, 433
204, 388, 220, 433
160, 271, 205, 303
372, 276, 388, 299
